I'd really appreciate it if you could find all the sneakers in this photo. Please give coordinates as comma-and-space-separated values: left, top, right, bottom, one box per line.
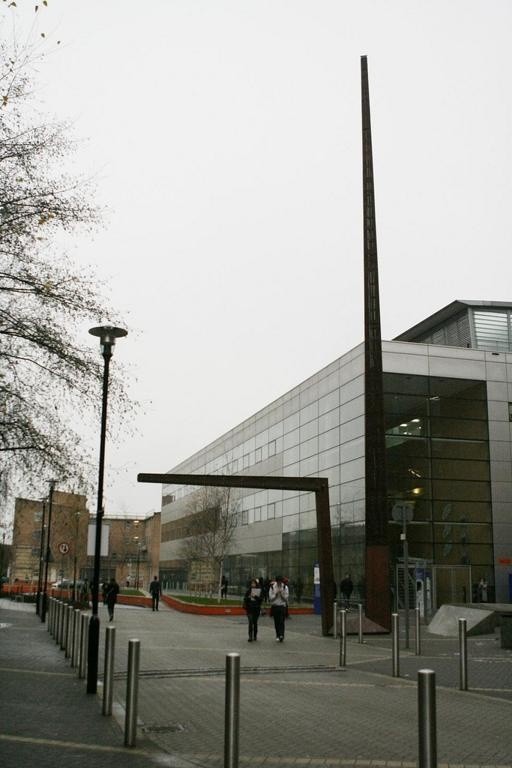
247, 634, 285, 643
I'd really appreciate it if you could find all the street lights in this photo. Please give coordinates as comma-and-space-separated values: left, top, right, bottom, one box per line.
87, 323, 130, 695
34, 493, 48, 615
40, 477, 61, 622
73, 511, 83, 601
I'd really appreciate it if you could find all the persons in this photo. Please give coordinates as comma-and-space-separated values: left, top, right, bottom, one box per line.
102, 578, 109, 605
81, 578, 90, 608
244, 577, 265, 642
476, 576, 489, 603
149, 575, 163, 612
220, 575, 228, 600
126, 574, 132, 587
107, 577, 120, 622
246, 574, 293, 620
268, 575, 289, 642
339, 571, 354, 609
